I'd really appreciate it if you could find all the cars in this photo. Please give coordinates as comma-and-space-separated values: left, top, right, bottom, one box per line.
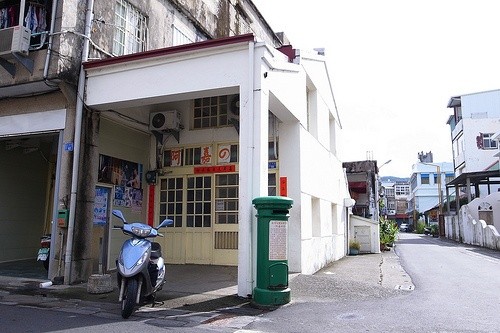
399, 223, 410, 232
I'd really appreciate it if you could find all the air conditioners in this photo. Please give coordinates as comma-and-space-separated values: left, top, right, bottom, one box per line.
227, 95, 240, 120
150, 110, 183, 130
0, 26, 31, 59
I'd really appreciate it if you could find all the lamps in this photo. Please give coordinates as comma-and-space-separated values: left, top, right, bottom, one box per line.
379, 160, 392, 169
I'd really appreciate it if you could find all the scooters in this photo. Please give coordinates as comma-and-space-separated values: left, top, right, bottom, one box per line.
36, 221, 53, 270
110, 209, 174, 320
